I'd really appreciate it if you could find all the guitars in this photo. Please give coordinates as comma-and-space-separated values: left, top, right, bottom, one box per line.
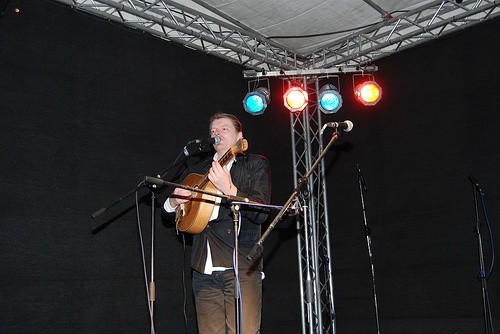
175, 139, 248, 234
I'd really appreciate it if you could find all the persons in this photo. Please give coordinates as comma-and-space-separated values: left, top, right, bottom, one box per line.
161, 114, 271, 334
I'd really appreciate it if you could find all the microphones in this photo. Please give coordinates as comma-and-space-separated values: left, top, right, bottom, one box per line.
357, 163, 367, 192
469, 175, 482, 193
325, 120, 354, 132
194, 136, 221, 145
